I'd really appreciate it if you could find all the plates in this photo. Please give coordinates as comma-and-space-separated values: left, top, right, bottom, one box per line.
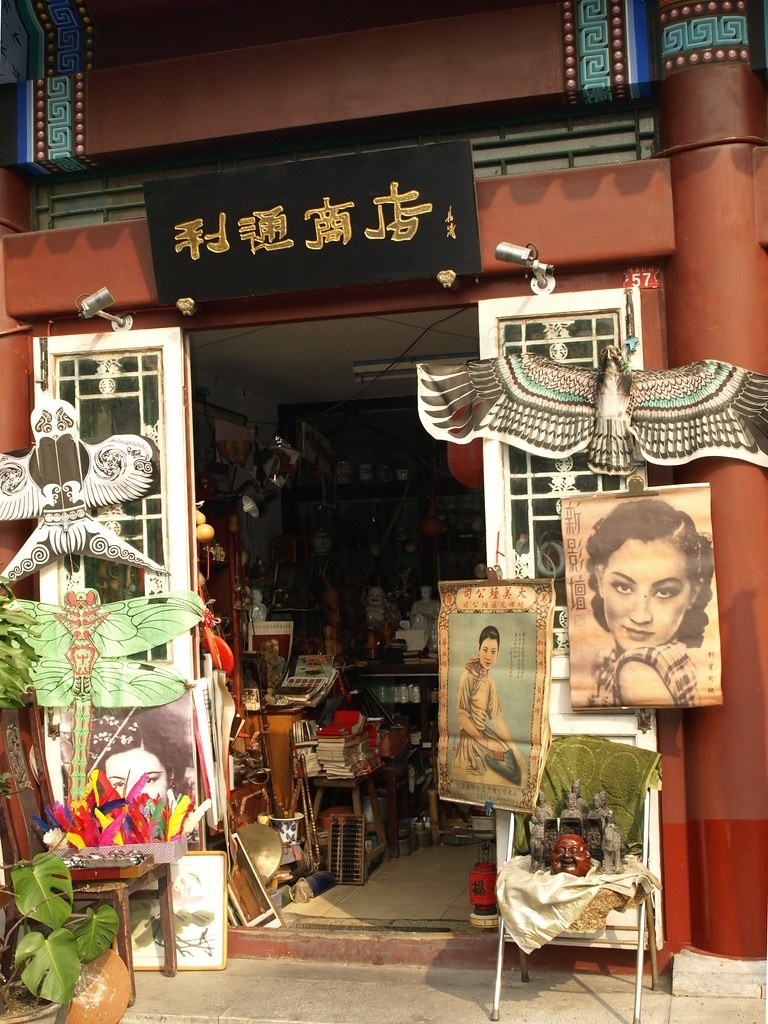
389, 643, 405, 648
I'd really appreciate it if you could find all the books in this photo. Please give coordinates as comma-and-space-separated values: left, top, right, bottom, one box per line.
293, 710, 382, 780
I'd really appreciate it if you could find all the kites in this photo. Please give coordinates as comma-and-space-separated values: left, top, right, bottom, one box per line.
415, 344, 768, 477
0, 588, 206, 810
0, 399, 171, 588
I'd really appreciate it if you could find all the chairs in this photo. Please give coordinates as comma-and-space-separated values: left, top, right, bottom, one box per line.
491, 772, 658, 1024
424, 724, 498, 846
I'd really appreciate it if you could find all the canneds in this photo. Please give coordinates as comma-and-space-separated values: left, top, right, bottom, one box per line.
377, 685, 421, 703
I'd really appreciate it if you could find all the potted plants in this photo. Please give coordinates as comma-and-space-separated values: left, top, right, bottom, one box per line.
0, 844, 119, 1024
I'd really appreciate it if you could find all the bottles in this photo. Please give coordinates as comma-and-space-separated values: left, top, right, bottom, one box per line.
415, 812, 425, 832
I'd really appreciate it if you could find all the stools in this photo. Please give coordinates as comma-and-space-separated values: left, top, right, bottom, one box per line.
302, 770, 390, 883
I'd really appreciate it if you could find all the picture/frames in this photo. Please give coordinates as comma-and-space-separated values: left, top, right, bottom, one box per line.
113, 850, 228, 971
206, 828, 287, 930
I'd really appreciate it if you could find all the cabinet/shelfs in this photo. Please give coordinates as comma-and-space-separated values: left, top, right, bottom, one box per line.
242, 665, 444, 859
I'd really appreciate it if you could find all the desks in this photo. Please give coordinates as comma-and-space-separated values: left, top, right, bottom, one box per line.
377, 755, 409, 858
51, 860, 178, 1008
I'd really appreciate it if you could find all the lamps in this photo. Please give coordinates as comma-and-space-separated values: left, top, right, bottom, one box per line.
75, 287, 133, 332
221, 479, 280, 519
494, 242, 557, 295
351, 352, 479, 384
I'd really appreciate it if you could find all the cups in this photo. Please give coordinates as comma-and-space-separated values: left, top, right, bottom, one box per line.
430, 690, 438, 703
371, 684, 421, 704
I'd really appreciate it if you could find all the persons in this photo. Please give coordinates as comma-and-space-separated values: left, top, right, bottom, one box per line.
530, 779, 618, 876
586, 496, 714, 705
363, 587, 401, 646
411, 585, 440, 615
